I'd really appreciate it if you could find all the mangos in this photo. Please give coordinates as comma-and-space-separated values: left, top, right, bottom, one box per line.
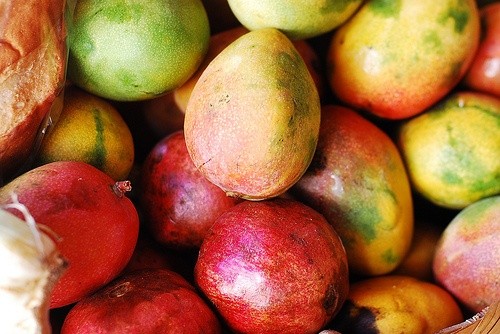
0, 0, 500, 334
